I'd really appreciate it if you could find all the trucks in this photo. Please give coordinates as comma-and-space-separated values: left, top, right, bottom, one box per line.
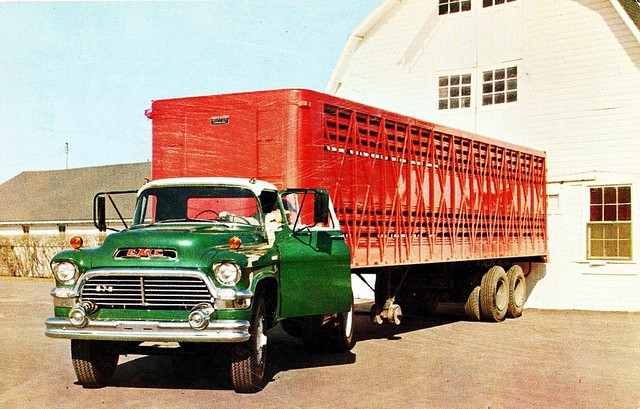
43, 85, 548, 393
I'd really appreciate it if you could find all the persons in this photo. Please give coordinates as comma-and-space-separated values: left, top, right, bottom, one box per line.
265, 199, 299, 225
